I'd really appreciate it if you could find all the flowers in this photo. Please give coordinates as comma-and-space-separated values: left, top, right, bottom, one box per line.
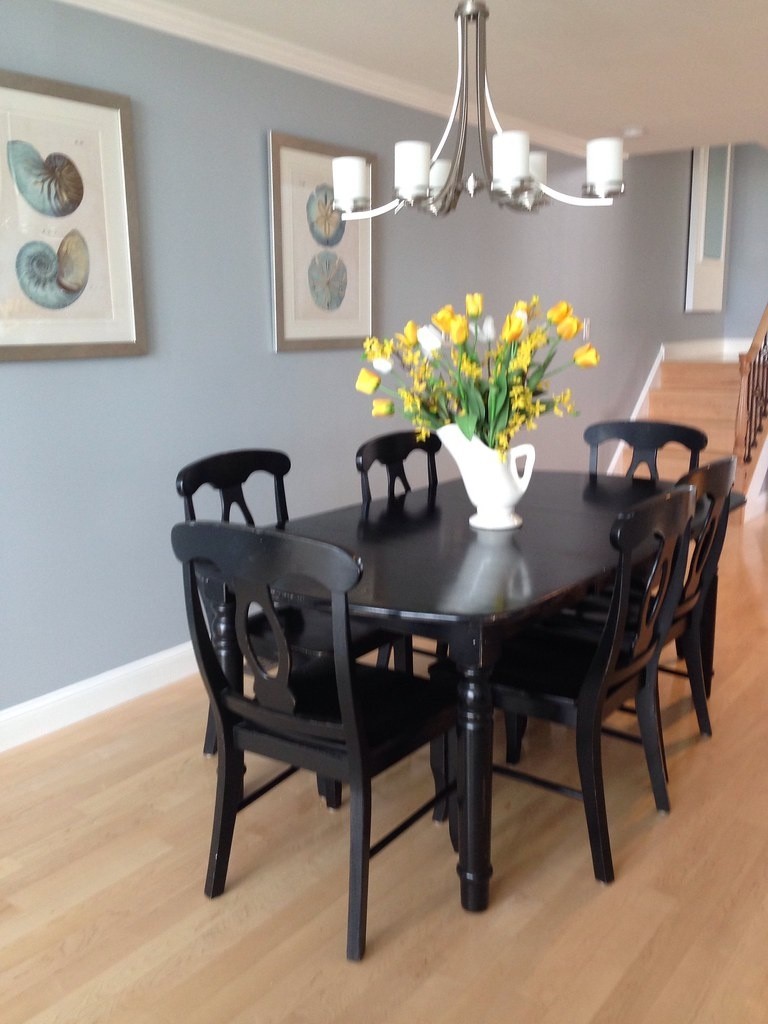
355, 292, 600, 468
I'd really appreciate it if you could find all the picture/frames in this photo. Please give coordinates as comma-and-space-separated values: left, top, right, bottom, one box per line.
0, 67, 149, 361
267, 129, 377, 354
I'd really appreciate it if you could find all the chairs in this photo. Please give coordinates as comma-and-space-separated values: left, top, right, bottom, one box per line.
564, 454, 742, 786
171, 519, 461, 961
357, 423, 440, 672
175, 447, 397, 812
427, 486, 698, 885
584, 419, 709, 481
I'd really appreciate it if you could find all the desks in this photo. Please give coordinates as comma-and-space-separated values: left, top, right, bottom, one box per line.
247, 471, 745, 912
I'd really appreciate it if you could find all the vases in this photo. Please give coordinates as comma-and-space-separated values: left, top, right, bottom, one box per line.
435, 422, 536, 530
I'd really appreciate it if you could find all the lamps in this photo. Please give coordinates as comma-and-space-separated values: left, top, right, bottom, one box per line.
332, 0, 626, 221
